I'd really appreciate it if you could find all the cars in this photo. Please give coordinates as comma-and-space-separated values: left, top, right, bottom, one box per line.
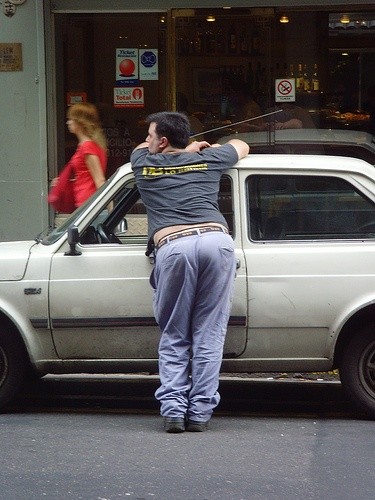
211, 128, 375, 169
0, 153, 375, 422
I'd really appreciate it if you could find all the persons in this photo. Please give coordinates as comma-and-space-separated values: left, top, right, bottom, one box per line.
275, 102, 324, 155
230, 79, 264, 130
50, 102, 113, 214
177, 92, 204, 141
130, 112, 250, 433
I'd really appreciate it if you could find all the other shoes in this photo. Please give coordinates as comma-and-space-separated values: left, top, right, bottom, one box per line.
164, 417, 185, 431
187, 418, 210, 431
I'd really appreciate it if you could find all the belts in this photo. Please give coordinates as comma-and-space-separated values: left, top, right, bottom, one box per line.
156, 227, 222, 252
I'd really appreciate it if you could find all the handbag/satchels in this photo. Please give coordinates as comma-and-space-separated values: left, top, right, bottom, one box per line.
49, 162, 74, 213
